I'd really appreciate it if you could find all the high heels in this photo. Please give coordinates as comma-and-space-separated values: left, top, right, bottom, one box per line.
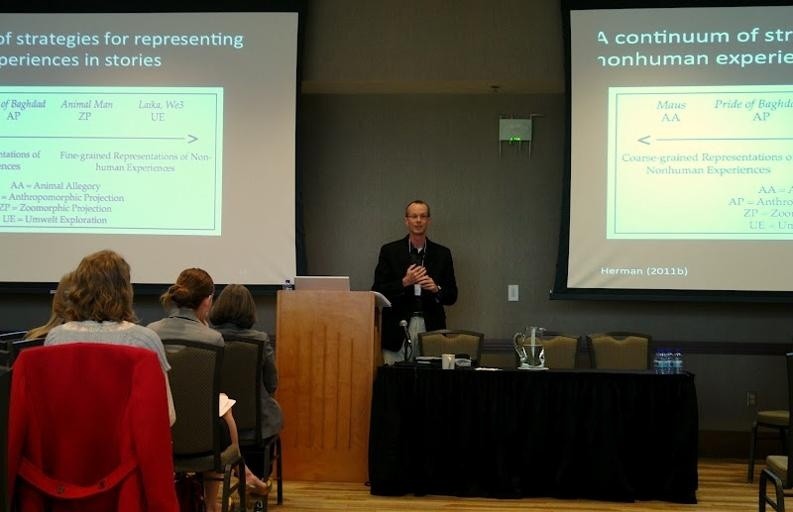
245, 479, 273, 506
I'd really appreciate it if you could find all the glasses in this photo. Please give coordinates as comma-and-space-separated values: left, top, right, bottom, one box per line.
406, 215, 429, 219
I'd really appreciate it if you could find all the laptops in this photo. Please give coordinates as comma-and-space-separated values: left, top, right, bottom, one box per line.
294, 277, 350, 293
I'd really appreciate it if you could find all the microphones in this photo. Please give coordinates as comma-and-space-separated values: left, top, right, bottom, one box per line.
400, 320, 412, 347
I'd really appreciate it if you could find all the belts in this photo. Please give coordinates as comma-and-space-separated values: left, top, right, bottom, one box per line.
412, 312, 424, 317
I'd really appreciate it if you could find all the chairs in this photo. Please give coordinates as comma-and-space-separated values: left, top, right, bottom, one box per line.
514, 332, 582, 369
747, 389, 791, 481
586, 332, 653, 371
758, 456, 793, 512
215, 333, 283, 504
22, 341, 161, 512
417, 329, 485, 364
153, 339, 247, 512
0, 331, 34, 343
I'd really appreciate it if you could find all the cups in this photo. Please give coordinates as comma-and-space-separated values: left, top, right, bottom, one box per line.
441, 354, 455, 369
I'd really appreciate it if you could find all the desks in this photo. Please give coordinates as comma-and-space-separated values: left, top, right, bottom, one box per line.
371, 361, 698, 505
276, 290, 374, 484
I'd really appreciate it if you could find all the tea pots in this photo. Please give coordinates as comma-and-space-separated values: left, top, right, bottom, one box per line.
513, 326, 550, 371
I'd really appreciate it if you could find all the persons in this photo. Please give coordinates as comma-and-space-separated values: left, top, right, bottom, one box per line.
23, 248, 176, 428
371, 200, 458, 365
207, 283, 285, 443
144, 267, 272, 512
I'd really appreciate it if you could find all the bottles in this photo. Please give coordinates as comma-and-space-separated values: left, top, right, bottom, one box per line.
654, 348, 684, 371
282, 280, 292, 291
